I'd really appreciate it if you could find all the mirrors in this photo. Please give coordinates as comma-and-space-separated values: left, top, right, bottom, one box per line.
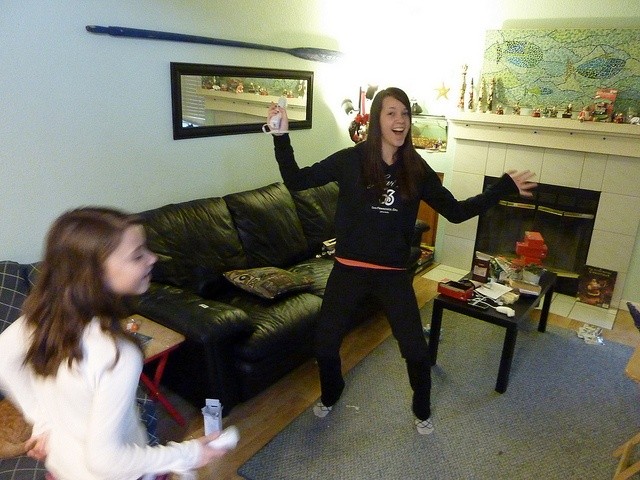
167, 59, 315, 140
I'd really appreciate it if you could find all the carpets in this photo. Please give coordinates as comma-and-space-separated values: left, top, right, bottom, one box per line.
236, 294, 639, 479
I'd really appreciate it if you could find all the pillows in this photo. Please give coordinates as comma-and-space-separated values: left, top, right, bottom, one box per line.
223, 265, 316, 298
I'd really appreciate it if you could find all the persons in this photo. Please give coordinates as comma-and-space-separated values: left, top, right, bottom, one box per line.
0, 204, 228, 480
265, 85, 541, 436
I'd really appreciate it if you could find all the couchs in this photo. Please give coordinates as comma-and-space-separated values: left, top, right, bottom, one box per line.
0, 260, 160, 480
123, 181, 431, 416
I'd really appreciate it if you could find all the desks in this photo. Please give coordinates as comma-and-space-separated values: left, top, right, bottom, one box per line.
117, 313, 188, 428
426, 254, 558, 396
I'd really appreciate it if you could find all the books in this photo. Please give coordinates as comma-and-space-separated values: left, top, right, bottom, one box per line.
477, 281, 513, 302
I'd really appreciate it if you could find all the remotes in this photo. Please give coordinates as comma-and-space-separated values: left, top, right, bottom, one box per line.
468, 301, 489, 310
